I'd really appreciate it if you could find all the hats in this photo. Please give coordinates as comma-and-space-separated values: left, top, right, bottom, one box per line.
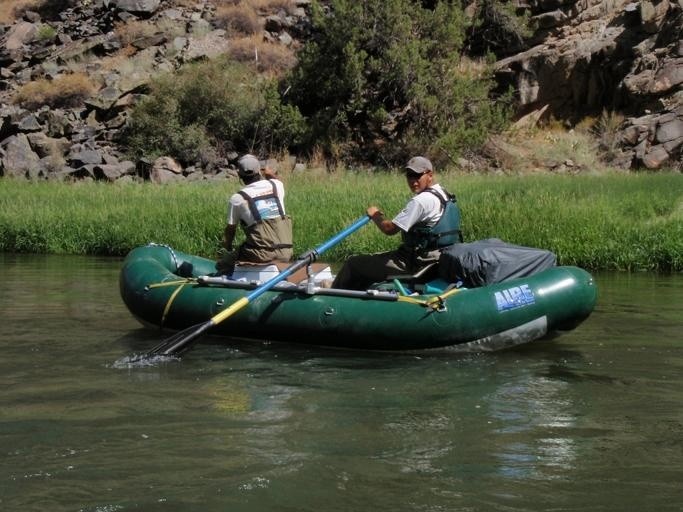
238, 154, 260, 176
398, 157, 432, 174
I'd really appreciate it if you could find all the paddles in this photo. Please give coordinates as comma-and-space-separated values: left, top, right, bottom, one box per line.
149, 215, 369, 358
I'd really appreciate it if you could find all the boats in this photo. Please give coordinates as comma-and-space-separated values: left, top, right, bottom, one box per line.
118, 242, 597, 357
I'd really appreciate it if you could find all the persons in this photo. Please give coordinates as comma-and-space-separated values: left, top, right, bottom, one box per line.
213, 154, 293, 272
330, 155, 461, 291
